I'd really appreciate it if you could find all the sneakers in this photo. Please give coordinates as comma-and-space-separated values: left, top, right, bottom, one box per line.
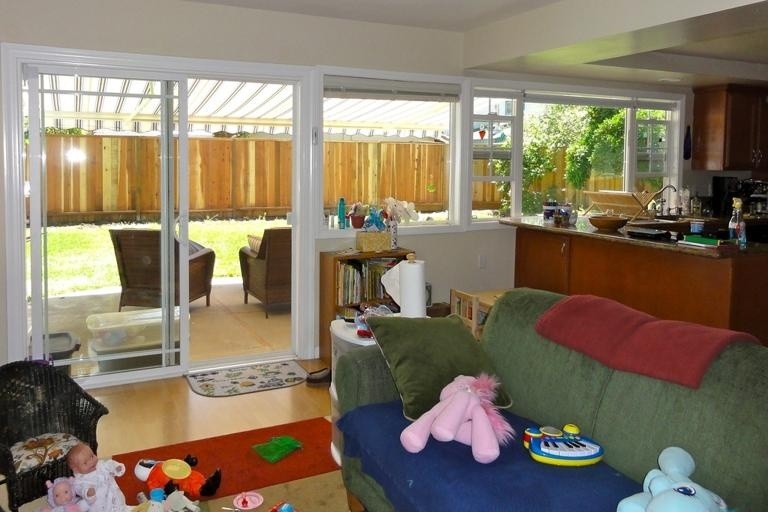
306, 367, 331, 383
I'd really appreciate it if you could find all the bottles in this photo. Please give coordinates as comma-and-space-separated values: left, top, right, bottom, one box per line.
543, 201, 578, 228
338, 198, 350, 229
669, 184, 702, 216
728, 208, 747, 250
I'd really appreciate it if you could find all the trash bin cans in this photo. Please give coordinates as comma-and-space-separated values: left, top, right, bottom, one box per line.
49, 331, 81, 377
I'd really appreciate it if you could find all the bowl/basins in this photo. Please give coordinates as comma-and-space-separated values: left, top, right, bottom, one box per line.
588, 216, 628, 232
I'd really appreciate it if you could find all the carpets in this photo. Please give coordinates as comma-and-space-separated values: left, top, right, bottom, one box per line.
109, 415, 342, 505
182, 358, 309, 398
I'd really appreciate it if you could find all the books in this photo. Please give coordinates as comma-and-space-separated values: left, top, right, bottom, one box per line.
336, 257, 399, 308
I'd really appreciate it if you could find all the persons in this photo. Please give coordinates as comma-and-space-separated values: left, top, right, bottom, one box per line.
66, 443, 128, 512
40, 479, 89, 512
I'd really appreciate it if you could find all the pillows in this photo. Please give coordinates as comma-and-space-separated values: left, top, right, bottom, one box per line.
248, 235, 262, 253
363, 314, 513, 422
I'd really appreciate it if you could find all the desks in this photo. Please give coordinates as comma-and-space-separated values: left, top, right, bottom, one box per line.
476, 288, 501, 314
326, 314, 434, 467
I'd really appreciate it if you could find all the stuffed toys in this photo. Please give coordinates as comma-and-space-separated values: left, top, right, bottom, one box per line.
345, 201, 392, 233
130, 454, 223, 512
398, 370, 517, 464
616, 446, 728, 512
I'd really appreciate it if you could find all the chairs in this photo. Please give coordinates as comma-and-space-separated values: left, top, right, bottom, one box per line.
240, 226, 291, 320
450, 289, 485, 338
110, 230, 215, 312
0, 359, 110, 512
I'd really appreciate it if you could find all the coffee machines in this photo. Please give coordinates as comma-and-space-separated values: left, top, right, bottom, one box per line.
713, 176, 738, 217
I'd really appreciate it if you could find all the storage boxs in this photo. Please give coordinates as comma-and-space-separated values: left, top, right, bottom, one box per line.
85, 306, 192, 374
31, 332, 81, 378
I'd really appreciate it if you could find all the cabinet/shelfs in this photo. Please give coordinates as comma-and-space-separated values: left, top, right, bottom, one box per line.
319, 249, 412, 367
691, 82, 768, 172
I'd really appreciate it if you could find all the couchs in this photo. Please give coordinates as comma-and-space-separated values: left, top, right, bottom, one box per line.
334, 287, 767, 511
